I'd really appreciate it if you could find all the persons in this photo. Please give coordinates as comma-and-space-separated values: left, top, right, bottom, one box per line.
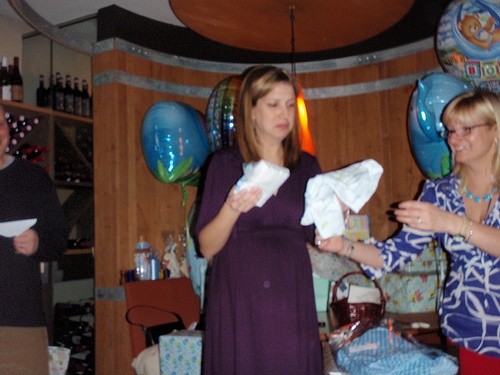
315, 88, 500, 375
194, 65, 349, 375
0, 104, 68, 374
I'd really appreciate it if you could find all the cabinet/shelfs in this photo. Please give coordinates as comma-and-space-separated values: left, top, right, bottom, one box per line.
0, 99, 93, 343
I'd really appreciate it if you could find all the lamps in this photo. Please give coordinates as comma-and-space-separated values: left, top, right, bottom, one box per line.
287, 8, 315, 155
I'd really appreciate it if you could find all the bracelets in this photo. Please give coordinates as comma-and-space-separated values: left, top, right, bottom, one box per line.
459, 215, 467, 236
336, 235, 354, 260
464, 219, 473, 243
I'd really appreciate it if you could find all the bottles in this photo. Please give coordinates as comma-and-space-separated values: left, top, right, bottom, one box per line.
52, 295, 96, 375
0, 54, 92, 119
4, 111, 93, 183
135, 235, 159, 281
67, 237, 94, 250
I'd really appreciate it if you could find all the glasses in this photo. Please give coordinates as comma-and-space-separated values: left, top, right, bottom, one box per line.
439, 124, 488, 139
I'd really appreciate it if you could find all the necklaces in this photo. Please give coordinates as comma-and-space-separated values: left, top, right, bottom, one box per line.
459, 181, 498, 203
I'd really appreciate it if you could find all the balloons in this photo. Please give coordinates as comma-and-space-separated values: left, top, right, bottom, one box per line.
407, 72, 476, 181
203, 73, 244, 154
141, 100, 210, 207
436, 0, 499, 93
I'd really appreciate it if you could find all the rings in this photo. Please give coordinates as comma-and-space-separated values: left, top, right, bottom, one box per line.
316, 240, 321, 247
416, 217, 422, 225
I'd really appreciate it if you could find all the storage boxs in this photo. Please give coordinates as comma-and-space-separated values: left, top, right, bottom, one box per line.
381, 245, 447, 313
159, 329, 203, 375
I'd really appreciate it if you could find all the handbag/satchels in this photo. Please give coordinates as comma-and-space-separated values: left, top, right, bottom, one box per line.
126, 305, 187, 349
337, 328, 459, 375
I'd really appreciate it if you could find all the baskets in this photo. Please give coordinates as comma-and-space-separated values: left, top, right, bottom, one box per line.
327, 270, 386, 333
